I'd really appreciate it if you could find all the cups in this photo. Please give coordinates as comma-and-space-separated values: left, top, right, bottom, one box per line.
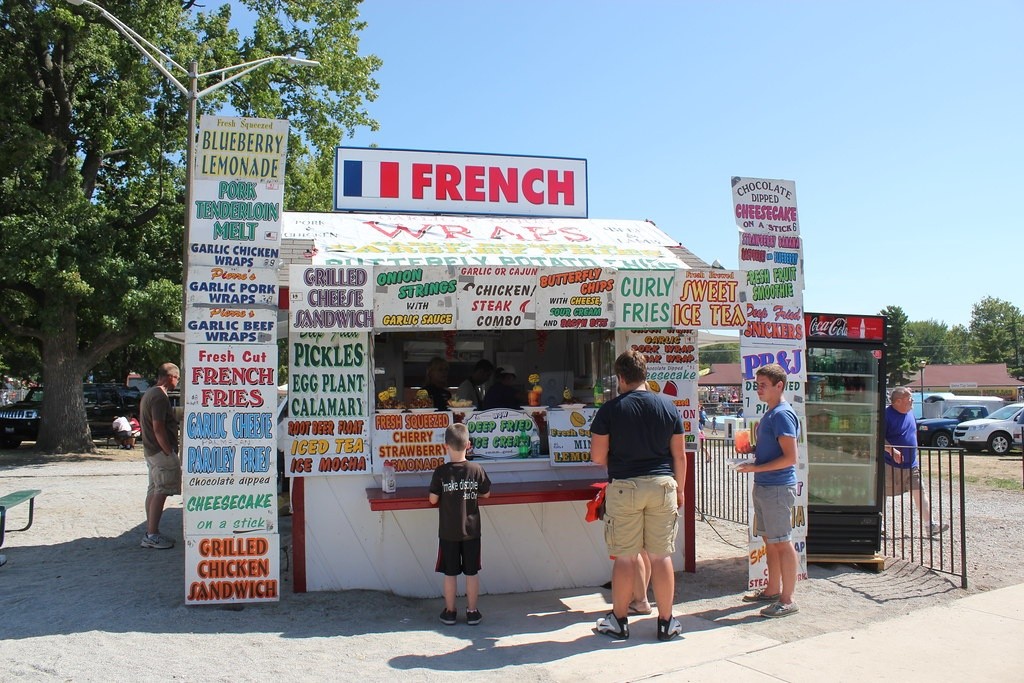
732, 429, 751, 452
528, 389, 541, 406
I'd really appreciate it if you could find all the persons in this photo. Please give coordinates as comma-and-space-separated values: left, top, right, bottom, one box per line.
457, 359, 494, 412
588, 351, 687, 642
735, 363, 801, 618
698, 406, 708, 426
737, 407, 743, 418
881, 387, 949, 539
717, 402, 729, 411
140, 363, 182, 549
480, 364, 525, 411
112, 412, 141, 450
711, 417, 718, 435
699, 422, 710, 463
732, 392, 737, 399
422, 357, 452, 411
429, 423, 492, 624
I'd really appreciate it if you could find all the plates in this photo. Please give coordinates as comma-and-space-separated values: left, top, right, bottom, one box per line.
408, 408, 438, 413
559, 404, 587, 408
374, 409, 405, 413
447, 406, 477, 411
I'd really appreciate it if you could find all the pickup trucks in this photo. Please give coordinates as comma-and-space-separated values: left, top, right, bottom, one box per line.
915, 405, 989, 447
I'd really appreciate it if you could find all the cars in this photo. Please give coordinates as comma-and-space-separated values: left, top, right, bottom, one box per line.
127, 373, 181, 430
953, 403, 1024, 456
1013, 409, 1024, 444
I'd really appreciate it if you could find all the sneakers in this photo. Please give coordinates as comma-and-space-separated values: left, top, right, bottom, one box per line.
439, 608, 457, 624
140, 531, 176, 549
760, 602, 800, 618
742, 590, 780, 602
658, 614, 682, 641
466, 606, 483, 624
596, 610, 629, 640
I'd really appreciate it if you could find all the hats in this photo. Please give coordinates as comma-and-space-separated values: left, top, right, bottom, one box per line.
497, 364, 517, 377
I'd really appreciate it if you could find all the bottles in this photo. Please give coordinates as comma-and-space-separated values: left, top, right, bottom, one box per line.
530, 429, 540, 457
519, 429, 528, 458
805, 376, 865, 403
808, 465, 867, 504
806, 406, 870, 434
594, 380, 604, 408
807, 347, 866, 373
381, 462, 396, 492
807, 435, 870, 463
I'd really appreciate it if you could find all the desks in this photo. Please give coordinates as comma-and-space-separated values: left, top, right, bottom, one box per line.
366, 477, 609, 515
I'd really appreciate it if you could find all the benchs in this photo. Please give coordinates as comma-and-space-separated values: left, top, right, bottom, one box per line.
93, 436, 138, 450
0, 489, 42, 546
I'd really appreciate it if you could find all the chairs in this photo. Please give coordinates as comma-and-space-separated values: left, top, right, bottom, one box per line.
969, 411, 981, 418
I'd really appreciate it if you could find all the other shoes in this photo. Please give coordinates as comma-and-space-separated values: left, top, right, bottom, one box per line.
119, 445, 130, 449
0, 554, 7, 567
925, 523, 949, 537
628, 599, 652, 616
881, 524, 885, 535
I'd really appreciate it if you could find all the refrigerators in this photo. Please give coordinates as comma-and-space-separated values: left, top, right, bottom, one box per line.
803, 313, 888, 554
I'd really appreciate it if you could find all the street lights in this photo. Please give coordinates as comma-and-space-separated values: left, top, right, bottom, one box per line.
916, 359, 927, 419
65, 0, 323, 469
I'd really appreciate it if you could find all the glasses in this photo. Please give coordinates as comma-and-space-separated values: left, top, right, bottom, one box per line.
167, 374, 180, 381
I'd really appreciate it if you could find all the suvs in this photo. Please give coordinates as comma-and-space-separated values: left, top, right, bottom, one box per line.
0, 383, 130, 449
